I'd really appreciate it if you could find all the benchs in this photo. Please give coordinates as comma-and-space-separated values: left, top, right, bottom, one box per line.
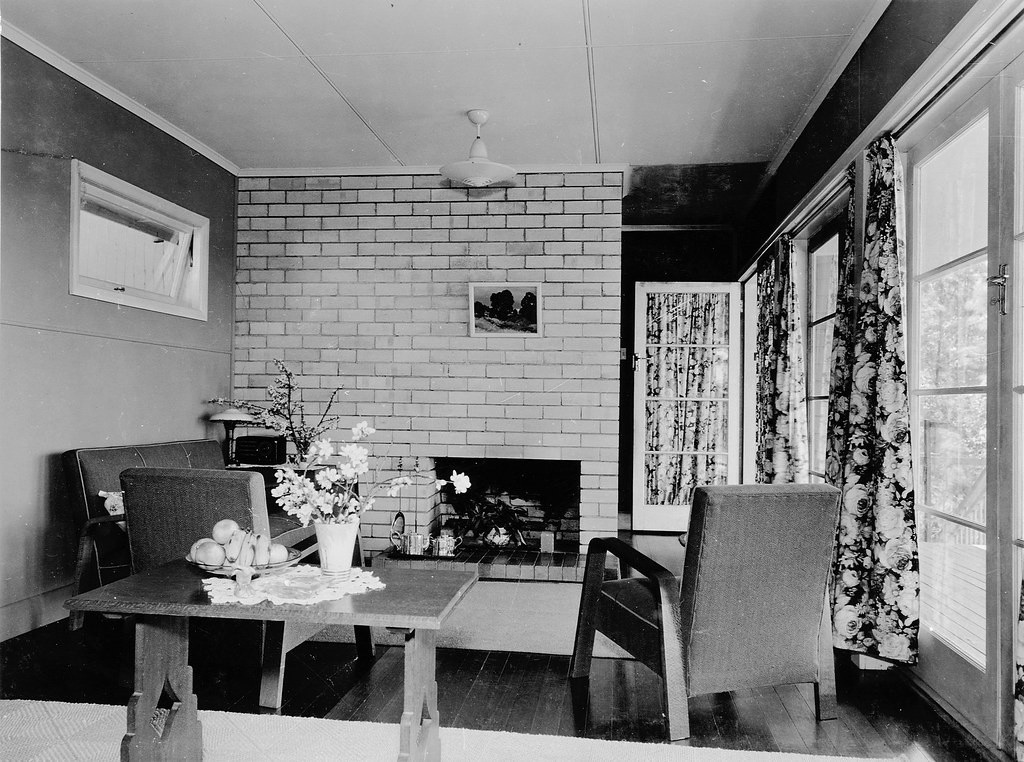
61, 438, 366, 631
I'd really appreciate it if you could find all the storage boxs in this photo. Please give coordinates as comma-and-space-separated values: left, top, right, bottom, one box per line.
235, 436, 286, 465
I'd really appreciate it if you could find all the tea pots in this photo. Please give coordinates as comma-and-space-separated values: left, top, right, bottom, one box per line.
390, 531, 432, 555
432, 535, 462, 556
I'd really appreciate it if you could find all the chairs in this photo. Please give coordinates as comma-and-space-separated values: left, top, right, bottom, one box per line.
118, 468, 377, 709
567, 482, 842, 742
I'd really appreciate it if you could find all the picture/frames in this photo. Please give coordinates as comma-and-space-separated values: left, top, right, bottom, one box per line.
468, 282, 543, 337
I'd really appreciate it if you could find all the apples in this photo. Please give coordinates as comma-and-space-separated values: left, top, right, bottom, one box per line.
191, 518, 288, 570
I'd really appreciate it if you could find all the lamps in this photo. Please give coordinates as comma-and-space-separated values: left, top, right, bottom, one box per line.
210, 408, 252, 466
439, 110, 517, 187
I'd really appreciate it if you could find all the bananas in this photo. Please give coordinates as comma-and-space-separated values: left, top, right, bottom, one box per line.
223, 527, 269, 569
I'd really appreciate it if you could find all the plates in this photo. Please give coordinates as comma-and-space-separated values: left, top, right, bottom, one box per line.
185, 548, 302, 576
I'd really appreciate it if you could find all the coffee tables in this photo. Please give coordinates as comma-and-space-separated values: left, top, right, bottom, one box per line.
60, 562, 480, 762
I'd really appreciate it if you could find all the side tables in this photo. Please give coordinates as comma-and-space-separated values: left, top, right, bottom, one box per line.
229, 464, 361, 532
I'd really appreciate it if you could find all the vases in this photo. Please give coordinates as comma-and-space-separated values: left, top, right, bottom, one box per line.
314, 518, 361, 581
298, 462, 310, 469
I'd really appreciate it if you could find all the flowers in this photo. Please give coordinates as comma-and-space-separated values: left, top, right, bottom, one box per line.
270, 421, 471, 521
209, 357, 351, 462
